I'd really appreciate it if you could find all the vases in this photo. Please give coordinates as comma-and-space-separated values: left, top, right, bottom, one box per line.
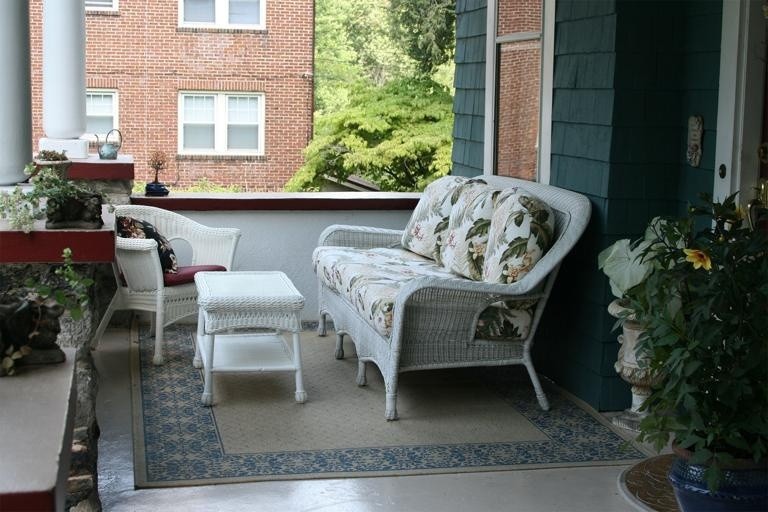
664, 435, 767, 511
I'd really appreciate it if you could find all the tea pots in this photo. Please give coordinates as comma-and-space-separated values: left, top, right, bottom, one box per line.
94, 129, 122, 159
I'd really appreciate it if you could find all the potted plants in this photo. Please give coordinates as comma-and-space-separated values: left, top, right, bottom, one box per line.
33, 150, 73, 180
141, 152, 183, 194
1, 247, 95, 376
1, 165, 116, 231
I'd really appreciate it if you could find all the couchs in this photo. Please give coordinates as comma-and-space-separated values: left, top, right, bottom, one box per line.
310, 174, 571, 420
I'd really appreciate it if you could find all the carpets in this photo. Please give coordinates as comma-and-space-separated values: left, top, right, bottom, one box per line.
618, 453, 682, 512
127, 320, 654, 492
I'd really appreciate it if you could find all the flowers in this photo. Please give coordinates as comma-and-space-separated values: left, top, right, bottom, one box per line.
612, 189, 767, 494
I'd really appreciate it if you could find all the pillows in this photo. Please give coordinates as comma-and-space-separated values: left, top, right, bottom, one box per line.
479, 187, 555, 306
440, 183, 516, 278
110, 215, 181, 273
401, 175, 484, 261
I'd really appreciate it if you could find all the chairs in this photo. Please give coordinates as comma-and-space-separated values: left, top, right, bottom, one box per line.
88, 204, 240, 369
